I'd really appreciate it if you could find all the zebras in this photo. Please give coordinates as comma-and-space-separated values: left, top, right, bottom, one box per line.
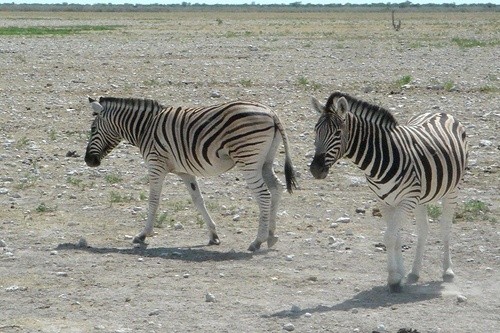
85, 96, 300, 253
310, 91, 468, 294
